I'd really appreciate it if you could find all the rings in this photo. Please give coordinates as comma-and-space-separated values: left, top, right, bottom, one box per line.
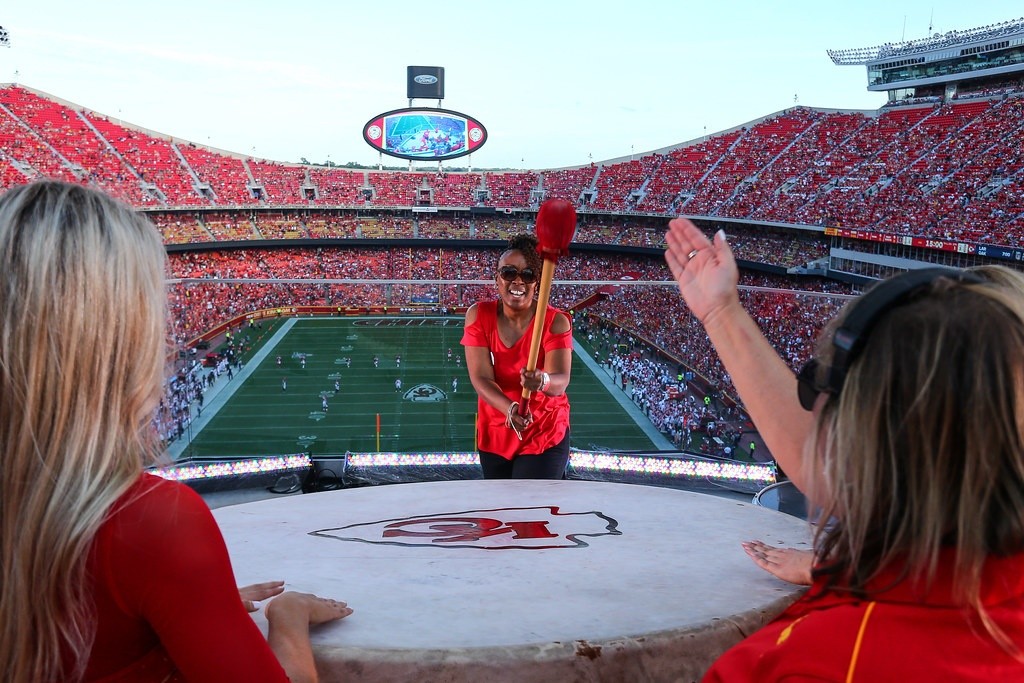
687, 248, 697, 259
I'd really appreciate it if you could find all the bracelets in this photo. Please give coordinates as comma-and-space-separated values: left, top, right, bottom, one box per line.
539, 371, 551, 393
507, 401, 520, 417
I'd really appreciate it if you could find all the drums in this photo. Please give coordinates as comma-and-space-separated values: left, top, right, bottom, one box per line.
210, 478, 826, 683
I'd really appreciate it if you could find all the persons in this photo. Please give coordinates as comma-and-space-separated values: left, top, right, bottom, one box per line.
461, 235, 574, 479
740, 538, 832, 586
2, 182, 356, 681
659, 214, 1024, 683
0, 84, 1023, 468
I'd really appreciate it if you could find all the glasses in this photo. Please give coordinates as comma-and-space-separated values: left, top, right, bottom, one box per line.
497, 266, 537, 284
795, 359, 821, 411
